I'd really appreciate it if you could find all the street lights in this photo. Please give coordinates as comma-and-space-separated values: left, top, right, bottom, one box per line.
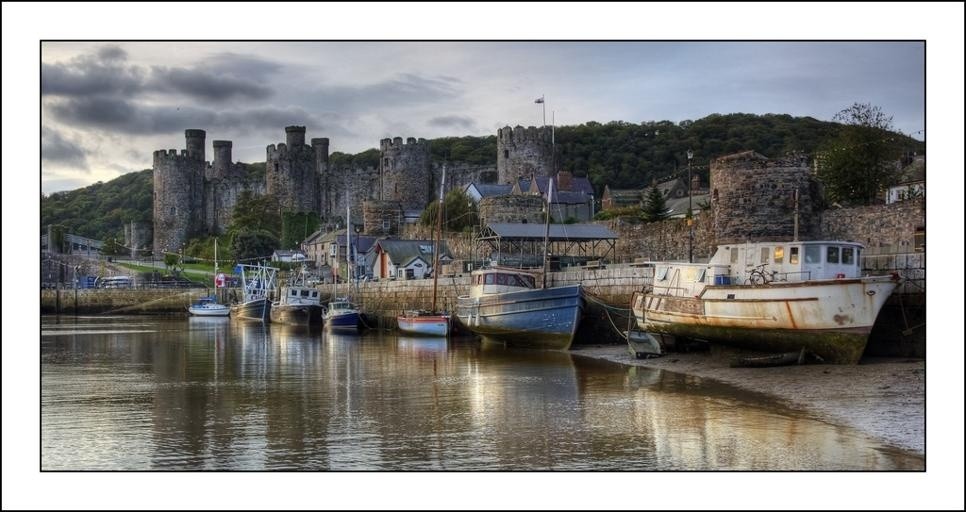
687, 149, 694, 263
535, 98, 545, 128
354, 225, 362, 284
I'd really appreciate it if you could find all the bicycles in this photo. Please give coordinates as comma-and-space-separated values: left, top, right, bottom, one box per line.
750, 264, 787, 285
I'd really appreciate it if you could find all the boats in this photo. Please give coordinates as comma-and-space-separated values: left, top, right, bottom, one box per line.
632, 241, 905, 365
457, 178, 586, 352
270, 287, 324, 326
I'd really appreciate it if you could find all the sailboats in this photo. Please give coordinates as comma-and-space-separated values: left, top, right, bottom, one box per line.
188, 239, 231, 316
230, 259, 280, 322
321, 207, 360, 332
398, 164, 452, 336
